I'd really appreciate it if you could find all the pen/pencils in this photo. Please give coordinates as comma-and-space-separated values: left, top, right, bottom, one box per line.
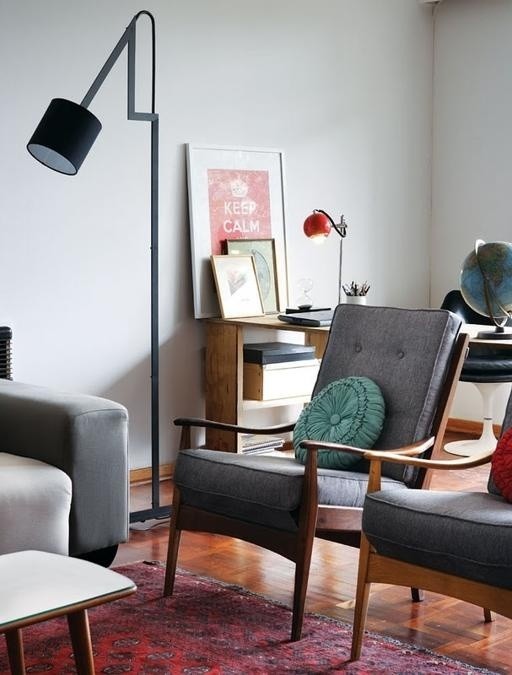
341, 280, 371, 296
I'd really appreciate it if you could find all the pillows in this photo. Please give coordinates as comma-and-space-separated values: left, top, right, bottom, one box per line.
491, 427, 512, 502
293, 378, 386, 470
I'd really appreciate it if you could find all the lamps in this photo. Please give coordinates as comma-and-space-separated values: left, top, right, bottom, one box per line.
24, 9, 175, 524
304, 206, 349, 309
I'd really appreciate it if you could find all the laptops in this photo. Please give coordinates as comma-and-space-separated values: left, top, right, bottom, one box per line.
277, 309, 334, 327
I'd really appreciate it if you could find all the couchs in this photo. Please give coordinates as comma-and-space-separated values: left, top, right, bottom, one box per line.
163, 304, 471, 643
351, 389, 512, 661
1, 370, 129, 567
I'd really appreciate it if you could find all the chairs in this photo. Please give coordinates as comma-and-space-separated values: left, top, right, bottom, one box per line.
441, 290, 512, 457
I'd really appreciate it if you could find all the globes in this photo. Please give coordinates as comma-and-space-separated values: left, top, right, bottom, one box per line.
459, 239, 512, 339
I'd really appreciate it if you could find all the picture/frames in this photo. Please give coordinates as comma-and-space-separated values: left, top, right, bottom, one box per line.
211, 254, 265, 320
185, 142, 290, 320
222, 237, 282, 316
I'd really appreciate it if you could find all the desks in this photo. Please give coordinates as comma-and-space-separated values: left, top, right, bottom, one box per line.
203, 319, 511, 455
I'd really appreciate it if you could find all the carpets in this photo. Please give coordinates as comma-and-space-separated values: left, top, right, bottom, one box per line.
0, 559, 496, 674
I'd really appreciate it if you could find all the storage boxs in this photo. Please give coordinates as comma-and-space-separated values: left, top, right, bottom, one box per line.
242, 360, 321, 400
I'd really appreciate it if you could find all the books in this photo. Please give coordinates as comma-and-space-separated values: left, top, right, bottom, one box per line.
242, 437, 285, 456
